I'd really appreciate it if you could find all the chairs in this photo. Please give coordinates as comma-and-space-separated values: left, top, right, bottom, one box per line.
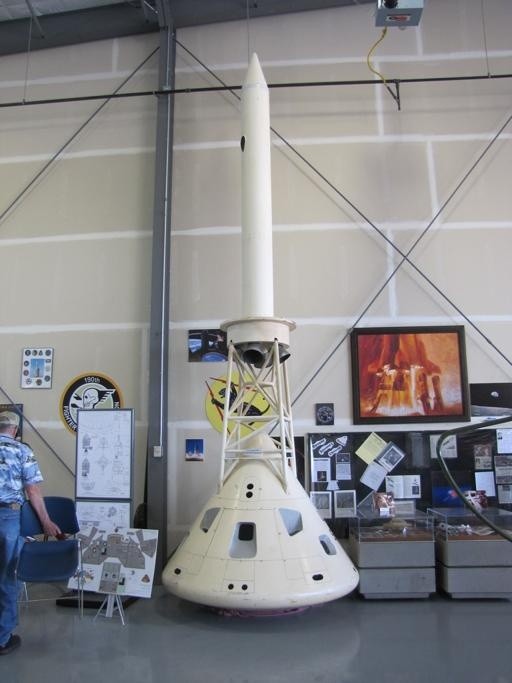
17, 497, 84, 621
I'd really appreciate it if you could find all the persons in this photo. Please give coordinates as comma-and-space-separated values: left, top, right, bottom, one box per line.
1, 409, 61, 655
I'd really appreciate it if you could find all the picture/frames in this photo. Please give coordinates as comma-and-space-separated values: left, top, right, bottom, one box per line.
350, 325, 471, 424
21, 348, 53, 389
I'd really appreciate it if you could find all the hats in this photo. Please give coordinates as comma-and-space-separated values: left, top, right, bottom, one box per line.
0, 411, 20, 426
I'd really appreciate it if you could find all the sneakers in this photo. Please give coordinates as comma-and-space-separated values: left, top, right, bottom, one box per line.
0, 635, 21, 654
